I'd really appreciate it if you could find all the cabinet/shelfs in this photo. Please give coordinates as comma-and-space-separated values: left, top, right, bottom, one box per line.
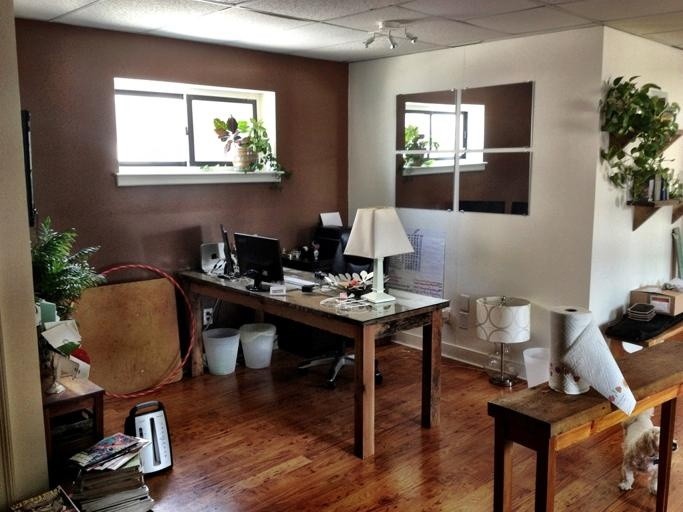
608, 124, 683, 232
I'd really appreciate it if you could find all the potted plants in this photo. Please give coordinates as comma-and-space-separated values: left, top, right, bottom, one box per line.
198, 113, 292, 192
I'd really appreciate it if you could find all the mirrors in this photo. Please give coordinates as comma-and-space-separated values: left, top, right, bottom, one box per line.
394, 81, 535, 219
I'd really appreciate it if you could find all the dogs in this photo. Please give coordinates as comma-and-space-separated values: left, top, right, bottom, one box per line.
617, 407, 678, 496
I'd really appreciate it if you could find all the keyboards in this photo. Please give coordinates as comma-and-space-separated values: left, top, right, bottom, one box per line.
283, 275, 320, 286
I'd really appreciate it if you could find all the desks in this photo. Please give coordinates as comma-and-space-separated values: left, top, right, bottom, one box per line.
488, 339, 683, 511
40, 363, 107, 492
178, 263, 451, 459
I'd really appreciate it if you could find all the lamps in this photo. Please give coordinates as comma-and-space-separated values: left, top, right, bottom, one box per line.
475, 294, 532, 389
342, 206, 415, 305
361, 20, 418, 48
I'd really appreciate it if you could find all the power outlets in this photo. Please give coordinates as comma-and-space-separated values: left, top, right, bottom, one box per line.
202, 307, 213, 326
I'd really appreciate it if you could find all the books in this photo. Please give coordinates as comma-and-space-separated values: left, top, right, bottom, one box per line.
7, 431, 154, 512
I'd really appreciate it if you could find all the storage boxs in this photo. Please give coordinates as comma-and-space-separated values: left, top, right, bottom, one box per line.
630, 286, 683, 317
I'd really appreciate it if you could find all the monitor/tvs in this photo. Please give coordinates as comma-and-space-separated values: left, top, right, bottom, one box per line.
234, 233, 283, 292
218, 224, 234, 279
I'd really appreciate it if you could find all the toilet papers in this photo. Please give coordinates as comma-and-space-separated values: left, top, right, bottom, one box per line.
548, 306, 637, 417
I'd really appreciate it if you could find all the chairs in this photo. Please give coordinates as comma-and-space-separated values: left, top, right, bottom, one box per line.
297, 232, 389, 389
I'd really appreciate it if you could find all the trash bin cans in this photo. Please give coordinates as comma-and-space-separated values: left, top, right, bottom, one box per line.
239, 323, 276, 369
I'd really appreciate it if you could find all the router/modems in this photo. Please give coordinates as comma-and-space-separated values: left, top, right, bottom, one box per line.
200, 242, 225, 273
